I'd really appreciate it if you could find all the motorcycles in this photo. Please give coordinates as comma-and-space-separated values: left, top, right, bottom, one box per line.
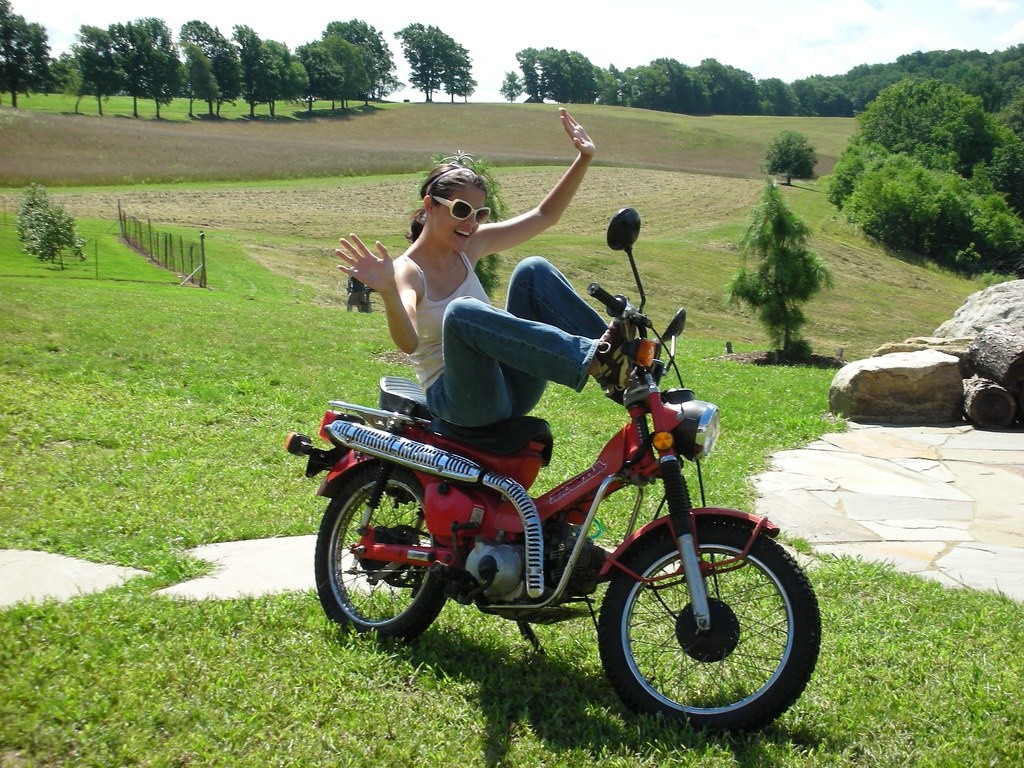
284, 205, 823, 737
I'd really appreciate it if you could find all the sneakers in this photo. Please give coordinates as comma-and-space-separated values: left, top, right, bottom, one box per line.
592, 309, 652, 408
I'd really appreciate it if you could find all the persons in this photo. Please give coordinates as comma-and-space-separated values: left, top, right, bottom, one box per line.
335, 108, 637, 427
346, 274, 364, 313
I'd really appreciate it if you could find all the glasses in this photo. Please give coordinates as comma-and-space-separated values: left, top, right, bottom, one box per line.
429, 192, 501, 224
